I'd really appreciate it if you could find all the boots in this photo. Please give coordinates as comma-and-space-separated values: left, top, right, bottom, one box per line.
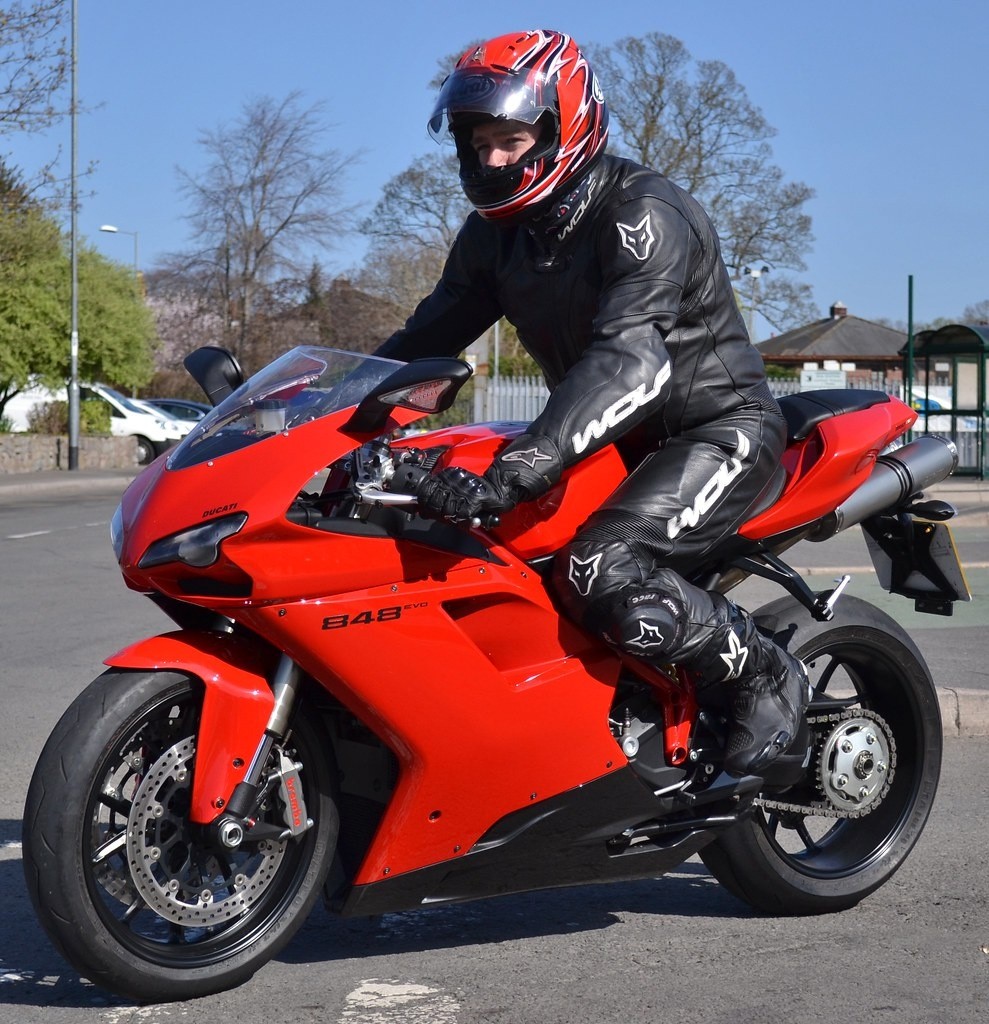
683, 591, 814, 779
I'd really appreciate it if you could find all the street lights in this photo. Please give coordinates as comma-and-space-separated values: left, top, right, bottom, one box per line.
746, 270, 762, 341
99, 224, 141, 281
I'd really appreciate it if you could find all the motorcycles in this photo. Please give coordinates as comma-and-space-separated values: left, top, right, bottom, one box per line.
19, 341, 972, 1005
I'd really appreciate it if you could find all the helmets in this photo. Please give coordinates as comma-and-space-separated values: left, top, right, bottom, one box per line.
427, 29, 610, 223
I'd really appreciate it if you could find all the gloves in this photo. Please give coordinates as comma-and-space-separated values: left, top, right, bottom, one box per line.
417, 432, 564, 533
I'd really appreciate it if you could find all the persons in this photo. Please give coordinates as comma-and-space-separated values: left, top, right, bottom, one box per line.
286, 27, 815, 782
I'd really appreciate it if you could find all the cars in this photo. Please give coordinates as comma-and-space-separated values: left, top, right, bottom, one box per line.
80, 380, 213, 466
